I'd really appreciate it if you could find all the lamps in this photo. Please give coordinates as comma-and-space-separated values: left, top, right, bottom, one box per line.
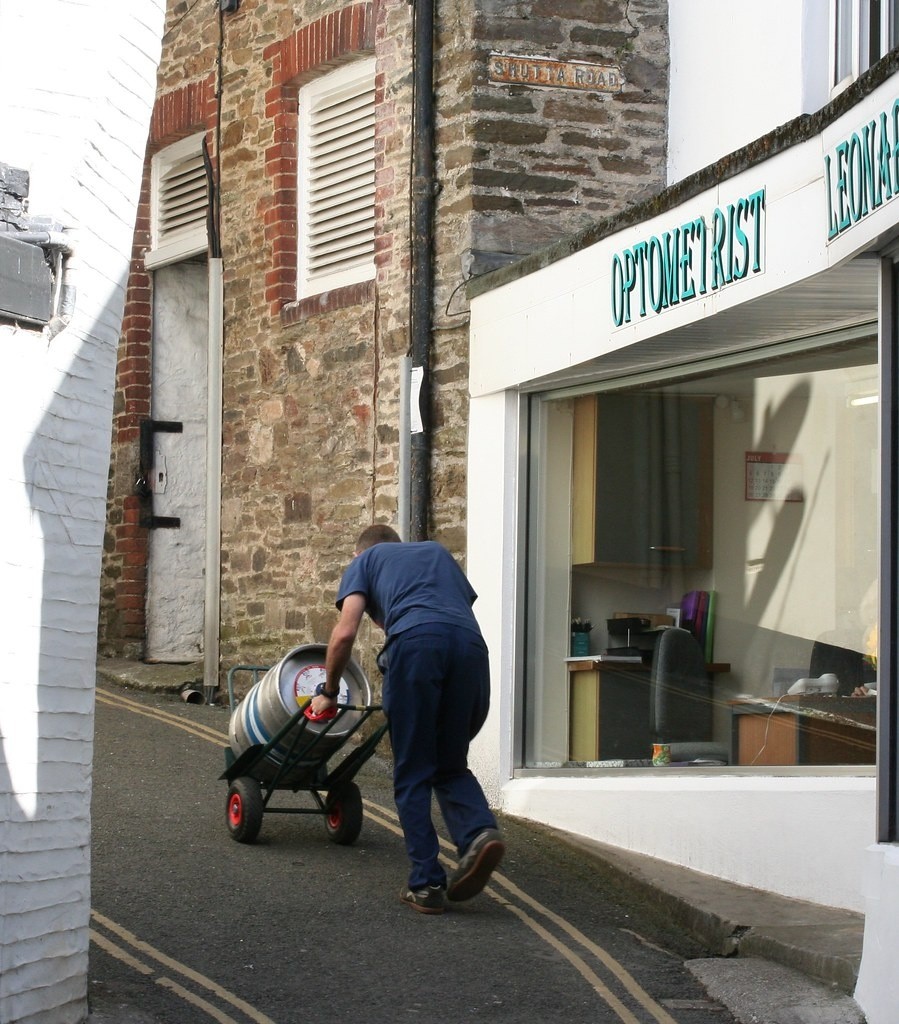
847, 392, 879, 410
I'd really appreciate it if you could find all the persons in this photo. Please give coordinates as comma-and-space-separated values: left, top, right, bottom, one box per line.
310, 525, 506, 913
807, 575, 880, 697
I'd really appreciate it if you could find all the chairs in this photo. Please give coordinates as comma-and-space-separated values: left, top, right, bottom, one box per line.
809, 631, 873, 695
649, 630, 729, 764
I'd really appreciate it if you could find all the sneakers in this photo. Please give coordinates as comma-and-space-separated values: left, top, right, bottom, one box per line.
399, 883, 445, 913
446, 828, 504, 902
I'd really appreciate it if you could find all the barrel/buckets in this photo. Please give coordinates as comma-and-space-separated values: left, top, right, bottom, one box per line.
229, 644, 372, 785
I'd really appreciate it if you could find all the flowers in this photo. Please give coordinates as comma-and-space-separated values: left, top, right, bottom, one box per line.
862, 624, 878, 671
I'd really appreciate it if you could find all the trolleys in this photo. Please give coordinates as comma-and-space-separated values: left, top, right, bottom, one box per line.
219, 665, 388, 846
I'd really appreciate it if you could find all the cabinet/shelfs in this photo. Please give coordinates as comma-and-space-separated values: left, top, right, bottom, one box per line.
568, 662, 731, 761
573, 391, 717, 571
728, 693, 877, 766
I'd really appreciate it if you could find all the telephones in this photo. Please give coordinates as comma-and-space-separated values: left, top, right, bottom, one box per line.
786, 671, 840, 699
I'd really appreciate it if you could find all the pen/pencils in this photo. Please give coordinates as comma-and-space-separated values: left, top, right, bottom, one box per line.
570, 614, 594, 637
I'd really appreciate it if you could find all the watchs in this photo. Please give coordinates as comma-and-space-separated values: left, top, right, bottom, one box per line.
315, 682, 340, 699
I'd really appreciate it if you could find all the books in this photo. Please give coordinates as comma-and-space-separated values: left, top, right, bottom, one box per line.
563, 654, 643, 664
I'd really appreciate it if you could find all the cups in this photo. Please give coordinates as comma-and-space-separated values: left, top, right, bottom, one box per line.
571, 632, 589, 656
653, 743, 672, 768
